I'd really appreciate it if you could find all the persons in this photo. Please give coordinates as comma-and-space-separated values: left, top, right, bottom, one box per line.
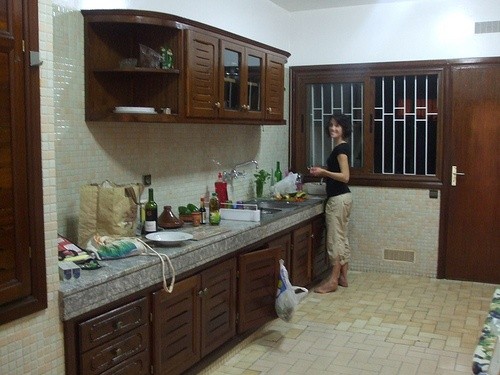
310, 113, 354, 293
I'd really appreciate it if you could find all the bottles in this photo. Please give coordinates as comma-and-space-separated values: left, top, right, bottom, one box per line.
274, 161, 282, 183
214, 172, 228, 208
157, 205, 180, 227
199, 198, 206, 224
209, 193, 221, 225
144, 188, 158, 234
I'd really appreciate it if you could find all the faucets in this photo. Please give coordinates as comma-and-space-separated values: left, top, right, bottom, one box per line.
223, 159, 259, 179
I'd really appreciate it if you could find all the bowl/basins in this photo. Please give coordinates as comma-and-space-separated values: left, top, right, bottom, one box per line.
303, 183, 326, 194
179, 214, 192, 221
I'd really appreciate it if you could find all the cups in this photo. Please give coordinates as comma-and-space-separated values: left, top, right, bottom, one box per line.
192, 212, 201, 226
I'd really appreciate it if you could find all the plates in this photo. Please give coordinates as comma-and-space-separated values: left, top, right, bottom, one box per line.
115, 106, 154, 112
145, 231, 193, 245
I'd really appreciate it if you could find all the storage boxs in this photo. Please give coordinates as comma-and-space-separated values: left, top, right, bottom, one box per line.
59, 260, 81, 281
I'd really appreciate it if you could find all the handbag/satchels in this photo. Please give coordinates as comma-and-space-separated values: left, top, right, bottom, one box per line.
274, 259, 308, 323
78, 179, 145, 249
87, 236, 146, 259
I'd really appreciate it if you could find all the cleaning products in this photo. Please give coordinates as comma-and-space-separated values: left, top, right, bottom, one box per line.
214, 171, 228, 208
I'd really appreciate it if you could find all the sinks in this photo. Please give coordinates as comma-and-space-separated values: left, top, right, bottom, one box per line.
249, 202, 298, 208
231, 208, 282, 214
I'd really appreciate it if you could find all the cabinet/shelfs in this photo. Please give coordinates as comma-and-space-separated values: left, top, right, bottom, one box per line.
80, 10, 291, 125
64, 213, 330, 375
